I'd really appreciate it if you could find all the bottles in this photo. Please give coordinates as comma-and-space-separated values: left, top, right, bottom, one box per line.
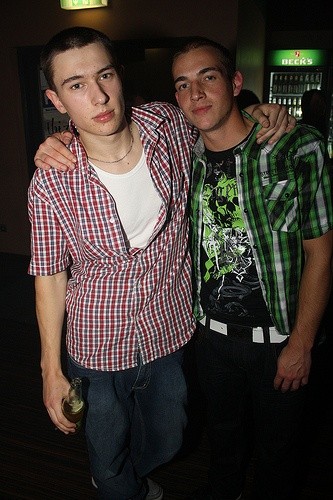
61, 377, 84, 431
271, 74, 320, 118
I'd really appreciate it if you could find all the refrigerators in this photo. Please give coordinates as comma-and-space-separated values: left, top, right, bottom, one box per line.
262, 50, 333, 151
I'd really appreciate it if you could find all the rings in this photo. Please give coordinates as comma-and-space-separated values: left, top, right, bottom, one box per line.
62, 130, 66, 134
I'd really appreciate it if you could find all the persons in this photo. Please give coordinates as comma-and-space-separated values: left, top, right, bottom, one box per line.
28, 27, 297, 500
297, 89, 328, 134
34, 38, 333, 500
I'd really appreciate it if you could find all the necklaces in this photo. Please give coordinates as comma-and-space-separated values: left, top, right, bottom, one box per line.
88, 134, 134, 163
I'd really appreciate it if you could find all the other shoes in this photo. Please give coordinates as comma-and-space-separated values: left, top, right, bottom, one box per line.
135, 475, 163, 500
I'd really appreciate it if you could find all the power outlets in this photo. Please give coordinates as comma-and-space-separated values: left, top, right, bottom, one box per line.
0, 224, 8, 232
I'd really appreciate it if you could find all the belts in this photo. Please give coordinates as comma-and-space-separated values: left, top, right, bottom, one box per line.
199, 316, 287, 343
